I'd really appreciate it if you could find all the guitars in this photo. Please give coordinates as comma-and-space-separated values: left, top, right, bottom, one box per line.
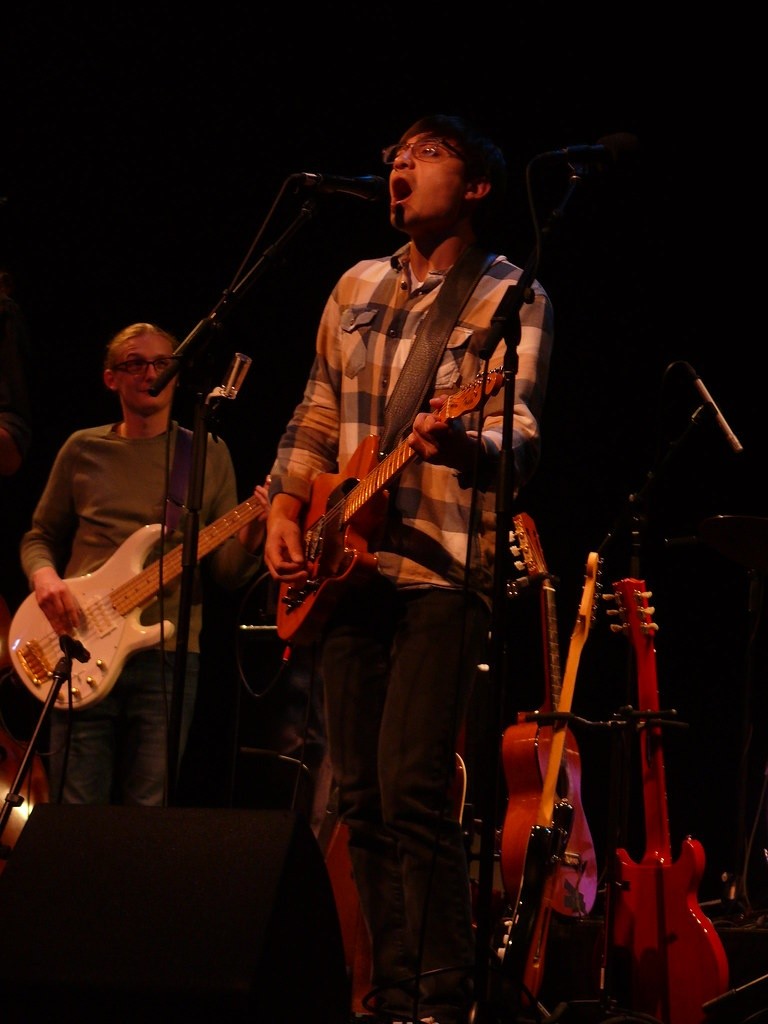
314, 746, 479, 1018
6, 472, 272, 712
499, 546, 604, 1017
496, 507, 601, 922
274, 363, 506, 647
593, 573, 733, 1024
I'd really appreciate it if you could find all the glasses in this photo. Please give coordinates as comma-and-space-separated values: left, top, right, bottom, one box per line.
112, 358, 170, 375
382, 144, 473, 164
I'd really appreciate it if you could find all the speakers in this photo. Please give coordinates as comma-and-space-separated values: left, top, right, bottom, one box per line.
0, 800, 352, 1024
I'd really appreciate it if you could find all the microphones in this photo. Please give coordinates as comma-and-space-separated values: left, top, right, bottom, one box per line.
60, 635, 90, 664
663, 360, 744, 459
299, 172, 390, 203
535, 133, 645, 172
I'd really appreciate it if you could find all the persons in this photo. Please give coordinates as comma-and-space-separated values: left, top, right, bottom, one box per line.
0, 274, 33, 477
265, 114, 556, 1024
22, 323, 266, 806
